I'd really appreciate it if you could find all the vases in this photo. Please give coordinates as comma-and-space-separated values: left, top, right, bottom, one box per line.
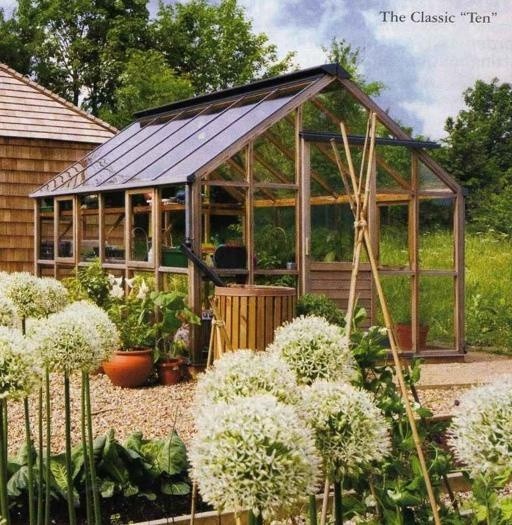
159, 358, 183, 385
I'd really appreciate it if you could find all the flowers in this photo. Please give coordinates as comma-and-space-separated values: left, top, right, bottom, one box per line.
157, 291, 202, 360
182, 315, 391, 523
0, 268, 122, 524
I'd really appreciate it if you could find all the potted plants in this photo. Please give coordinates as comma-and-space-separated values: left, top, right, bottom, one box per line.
100, 295, 157, 387
391, 283, 431, 351
